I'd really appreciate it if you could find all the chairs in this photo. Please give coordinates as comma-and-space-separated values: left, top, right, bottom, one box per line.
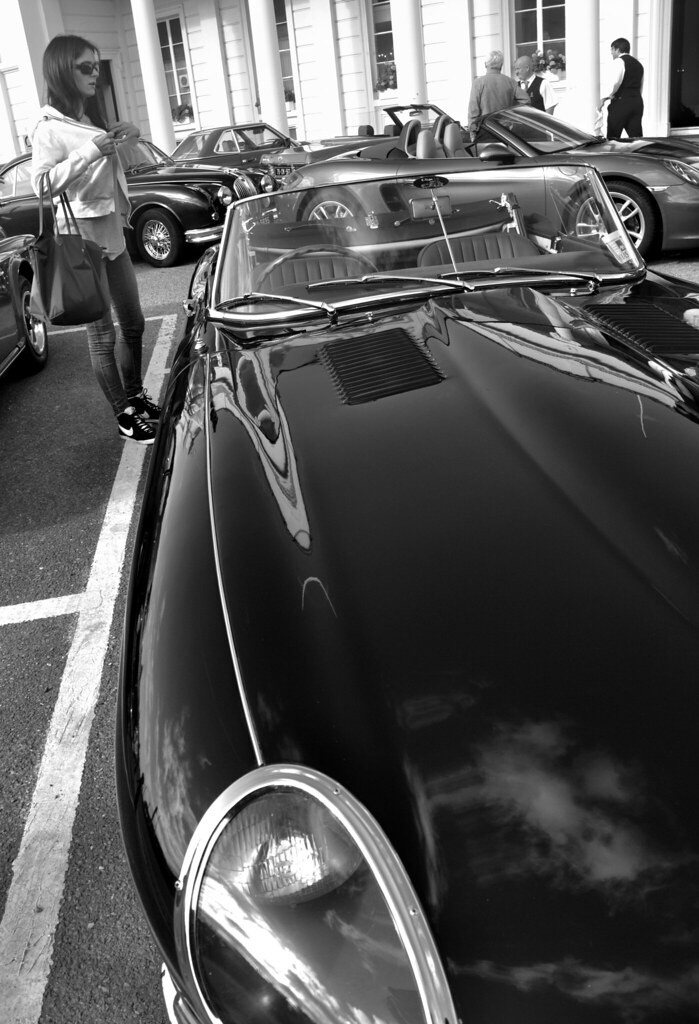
417, 232, 539, 267
443, 123, 473, 157
249, 255, 373, 293
384, 124, 399, 137
358, 125, 375, 137
222, 140, 245, 152
415, 130, 437, 159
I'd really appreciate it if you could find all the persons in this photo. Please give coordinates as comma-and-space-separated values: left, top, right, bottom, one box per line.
511, 55, 560, 116
597, 37, 644, 142
28, 35, 164, 445
468, 51, 529, 143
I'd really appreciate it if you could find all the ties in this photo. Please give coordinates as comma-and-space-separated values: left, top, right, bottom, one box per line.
521, 81, 529, 90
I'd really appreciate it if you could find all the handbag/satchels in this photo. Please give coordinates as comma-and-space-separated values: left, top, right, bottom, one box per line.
26, 168, 112, 328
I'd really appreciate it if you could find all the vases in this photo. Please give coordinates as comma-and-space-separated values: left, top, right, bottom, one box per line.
379, 87, 397, 99
173, 116, 193, 126
535, 68, 562, 82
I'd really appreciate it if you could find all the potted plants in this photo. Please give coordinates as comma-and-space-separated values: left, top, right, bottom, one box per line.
255, 89, 295, 112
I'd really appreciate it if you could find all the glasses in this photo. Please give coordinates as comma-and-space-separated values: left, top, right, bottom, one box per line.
73, 61, 100, 75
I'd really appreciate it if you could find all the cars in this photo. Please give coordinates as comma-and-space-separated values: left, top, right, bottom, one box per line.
158, 101, 699, 259
0, 135, 278, 268
115, 164, 699, 1023
0, 227, 49, 379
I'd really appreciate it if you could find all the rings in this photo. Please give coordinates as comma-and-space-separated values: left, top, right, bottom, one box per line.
124, 130, 128, 133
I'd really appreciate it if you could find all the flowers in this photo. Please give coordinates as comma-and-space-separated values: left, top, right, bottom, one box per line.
532, 50, 565, 73
376, 61, 396, 91
172, 104, 193, 122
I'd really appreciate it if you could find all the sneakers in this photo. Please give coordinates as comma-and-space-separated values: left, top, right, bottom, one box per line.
127, 388, 163, 424
116, 407, 158, 445
